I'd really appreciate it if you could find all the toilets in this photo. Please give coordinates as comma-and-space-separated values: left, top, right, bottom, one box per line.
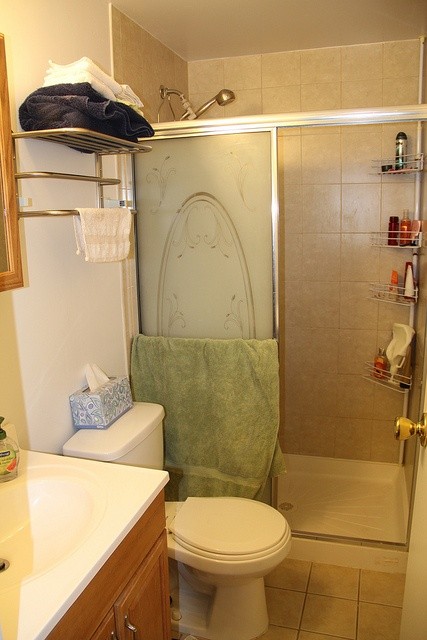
62, 399, 291, 640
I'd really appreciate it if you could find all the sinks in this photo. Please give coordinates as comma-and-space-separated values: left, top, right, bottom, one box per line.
0, 442, 169, 640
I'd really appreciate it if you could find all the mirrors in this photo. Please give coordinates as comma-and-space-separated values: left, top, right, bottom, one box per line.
0, 34, 24, 292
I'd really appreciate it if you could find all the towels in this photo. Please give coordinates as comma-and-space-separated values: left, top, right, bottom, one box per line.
19, 82, 154, 153
129, 336, 289, 503
72, 207, 132, 263
47, 56, 121, 96
116, 84, 144, 118
42, 71, 115, 102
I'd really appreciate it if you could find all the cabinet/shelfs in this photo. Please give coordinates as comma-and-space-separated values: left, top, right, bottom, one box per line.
364, 153, 423, 394
13, 128, 151, 219
46, 488, 172, 640
